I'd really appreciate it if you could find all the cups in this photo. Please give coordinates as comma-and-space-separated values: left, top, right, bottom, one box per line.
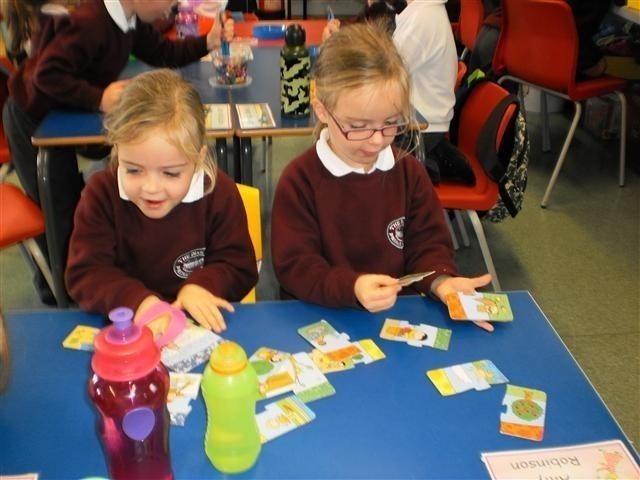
210, 42, 255, 85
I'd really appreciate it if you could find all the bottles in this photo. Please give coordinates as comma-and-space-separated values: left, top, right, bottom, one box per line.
199, 337, 264, 474
173, 0, 199, 39
278, 21, 313, 121
86, 302, 188, 479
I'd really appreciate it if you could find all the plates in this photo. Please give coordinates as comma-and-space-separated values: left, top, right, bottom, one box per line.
252, 24, 286, 40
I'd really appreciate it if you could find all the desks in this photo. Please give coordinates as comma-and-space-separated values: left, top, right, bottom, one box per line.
2, 290, 640, 475
28, 37, 426, 192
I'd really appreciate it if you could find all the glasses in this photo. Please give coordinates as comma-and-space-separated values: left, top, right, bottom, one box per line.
320, 97, 410, 142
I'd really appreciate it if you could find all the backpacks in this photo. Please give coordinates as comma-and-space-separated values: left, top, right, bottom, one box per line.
449, 76, 531, 225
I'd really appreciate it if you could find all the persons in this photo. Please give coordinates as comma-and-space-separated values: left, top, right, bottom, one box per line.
1, 1, 69, 73
3, 1, 235, 308
64, 69, 261, 351
271, 16, 496, 332
320, 0, 479, 187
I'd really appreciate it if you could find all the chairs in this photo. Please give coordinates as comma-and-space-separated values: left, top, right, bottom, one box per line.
456, 0, 628, 211
1, 134, 60, 307
434, 57, 511, 292
